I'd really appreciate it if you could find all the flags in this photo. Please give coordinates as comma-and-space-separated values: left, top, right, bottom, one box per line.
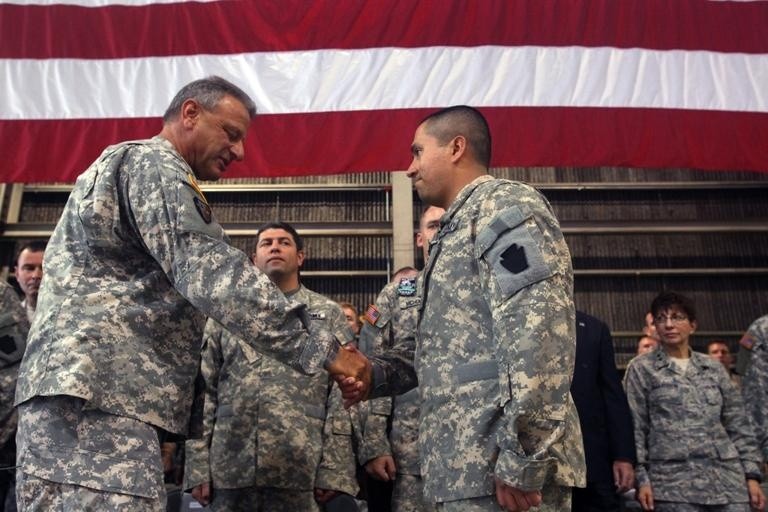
0, 0, 768, 189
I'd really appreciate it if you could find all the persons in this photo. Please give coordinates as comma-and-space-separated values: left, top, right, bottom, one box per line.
334, 104, 586, 511
0, 240, 52, 511
567, 289, 768, 512
14, 75, 370, 511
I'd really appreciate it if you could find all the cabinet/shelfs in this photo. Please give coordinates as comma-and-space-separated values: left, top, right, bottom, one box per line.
0, 162, 768, 388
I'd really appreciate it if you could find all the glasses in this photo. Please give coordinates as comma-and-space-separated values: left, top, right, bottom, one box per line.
653, 314, 687, 322
